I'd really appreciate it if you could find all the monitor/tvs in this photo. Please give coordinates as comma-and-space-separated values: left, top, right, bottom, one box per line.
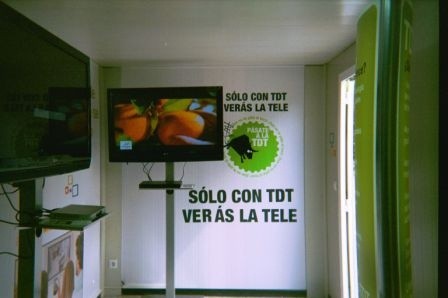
106, 85, 225, 161
0, 2, 92, 185
